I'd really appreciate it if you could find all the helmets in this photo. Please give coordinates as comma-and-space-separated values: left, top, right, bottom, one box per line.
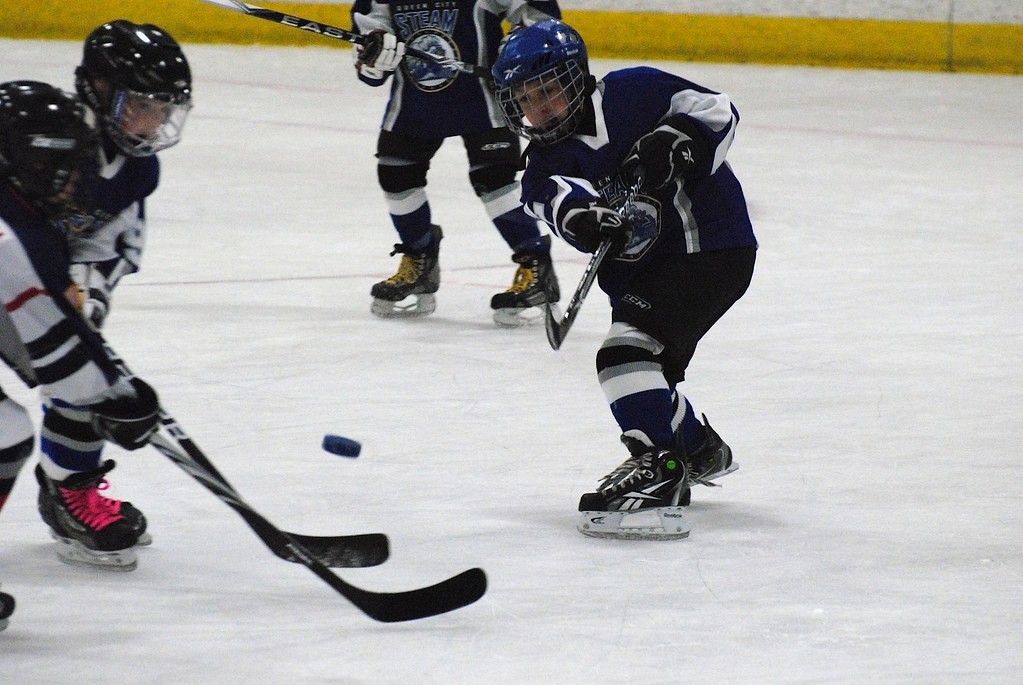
0, 80, 99, 218
74, 19, 193, 158
490, 18, 589, 147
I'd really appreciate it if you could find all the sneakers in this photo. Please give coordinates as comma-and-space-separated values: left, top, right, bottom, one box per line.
577, 423, 691, 542
35, 459, 153, 572
370, 224, 443, 320
685, 413, 740, 489
490, 234, 560, 328
0, 591, 15, 631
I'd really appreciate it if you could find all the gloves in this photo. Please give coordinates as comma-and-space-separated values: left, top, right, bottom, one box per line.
89, 377, 159, 450
619, 121, 696, 195
69, 264, 109, 330
556, 196, 634, 259
356, 28, 404, 79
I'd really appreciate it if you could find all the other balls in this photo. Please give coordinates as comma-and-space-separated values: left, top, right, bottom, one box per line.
321, 435, 364, 459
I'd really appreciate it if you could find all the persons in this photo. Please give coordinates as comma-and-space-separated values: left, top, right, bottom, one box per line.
0, 80, 159, 630
34, 20, 192, 566
493, 19, 758, 544
350, 0, 563, 329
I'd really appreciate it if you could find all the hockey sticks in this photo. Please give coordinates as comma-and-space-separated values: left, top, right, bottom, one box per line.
85, 321, 391, 568
544, 174, 643, 352
149, 436, 489, 627
206, 1, 493, 83
